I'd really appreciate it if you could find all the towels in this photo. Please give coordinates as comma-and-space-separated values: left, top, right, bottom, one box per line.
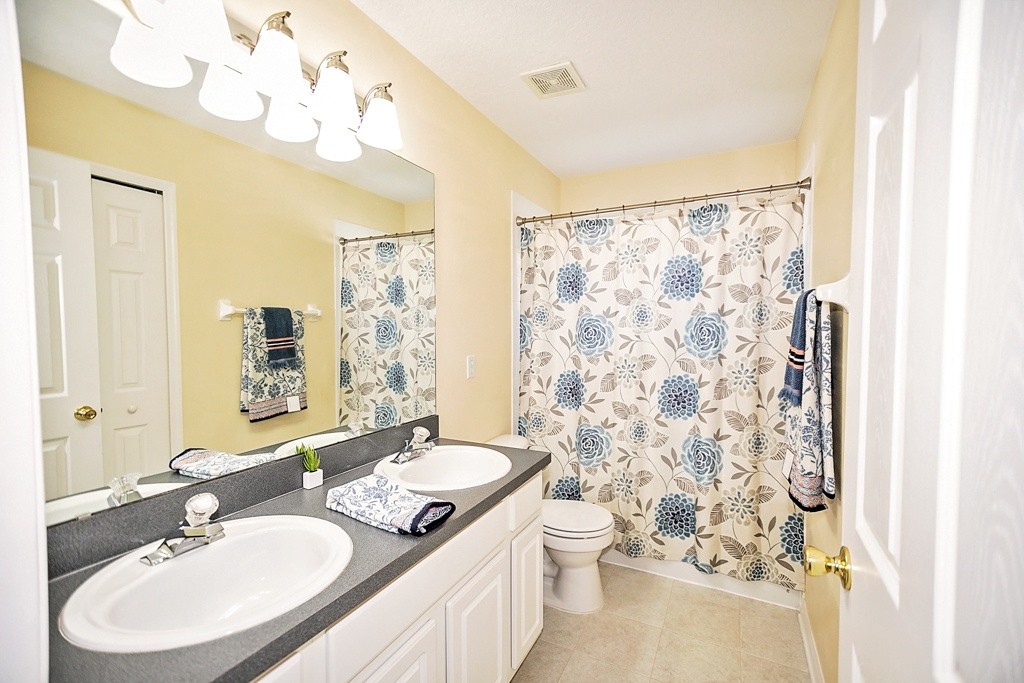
785, 293, 840, 512
261, 306, 297, 362
240, 306, 309, 422
778, 288, 815, 405
168, 446, 276, 480
324, 473, 456, 536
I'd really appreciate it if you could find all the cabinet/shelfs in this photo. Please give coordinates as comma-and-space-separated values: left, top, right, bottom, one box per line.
248, 469, 547, 683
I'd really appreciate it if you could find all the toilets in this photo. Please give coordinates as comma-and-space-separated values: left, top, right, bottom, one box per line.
482, 433, 617, 615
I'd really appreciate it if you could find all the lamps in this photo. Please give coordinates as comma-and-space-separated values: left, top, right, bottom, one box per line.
108, 17, 364, 163
148, 0, 403, 153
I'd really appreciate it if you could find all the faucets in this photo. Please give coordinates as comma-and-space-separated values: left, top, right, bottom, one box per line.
344, 419, 365, 438
105, 475, 142, 506
138, 491, 226, 566
390, 426, 436, 464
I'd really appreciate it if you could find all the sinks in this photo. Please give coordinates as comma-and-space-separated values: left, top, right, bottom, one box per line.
57, 515, 356, 654
375, 444, 512, 493
45, 482, 189, 528
276, 431, 349, 459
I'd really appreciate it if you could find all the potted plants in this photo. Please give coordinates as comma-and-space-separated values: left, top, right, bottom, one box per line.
302, 444, 324, 490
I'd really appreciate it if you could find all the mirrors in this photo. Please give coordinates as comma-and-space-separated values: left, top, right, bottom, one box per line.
14, 0, 437, 534
